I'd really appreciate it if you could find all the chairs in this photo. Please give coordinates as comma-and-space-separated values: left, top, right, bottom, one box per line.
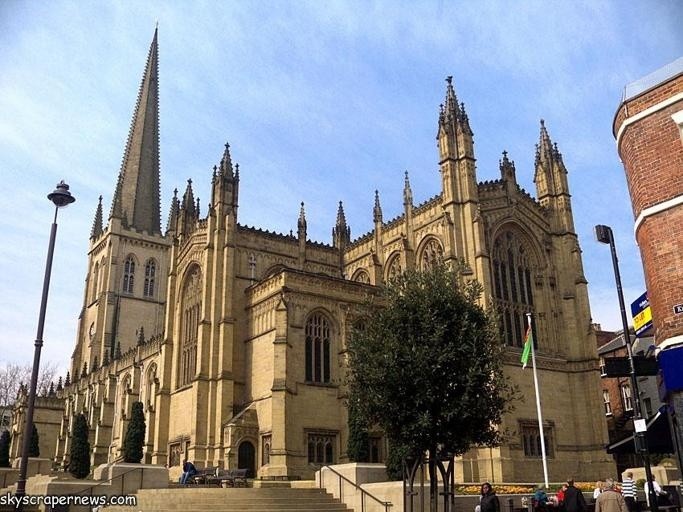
179, 467, 248, 488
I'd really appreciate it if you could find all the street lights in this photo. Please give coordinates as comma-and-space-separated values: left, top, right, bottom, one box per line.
594, 224, 658, 512
15, 180, 76, 510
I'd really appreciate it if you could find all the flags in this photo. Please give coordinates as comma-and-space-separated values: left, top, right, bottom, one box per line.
520, 316, 533, 371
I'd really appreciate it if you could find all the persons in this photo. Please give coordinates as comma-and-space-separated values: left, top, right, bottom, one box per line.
480, 483, 500, 512
620, 472, 638, 512
595, 478, 629, 512
179, 458, 198, 484
558, 477, 587, 512
644, 474, 667, 512
593, 481, 605, 500
534, 482, 549, 512
556, 484, 569, 504
